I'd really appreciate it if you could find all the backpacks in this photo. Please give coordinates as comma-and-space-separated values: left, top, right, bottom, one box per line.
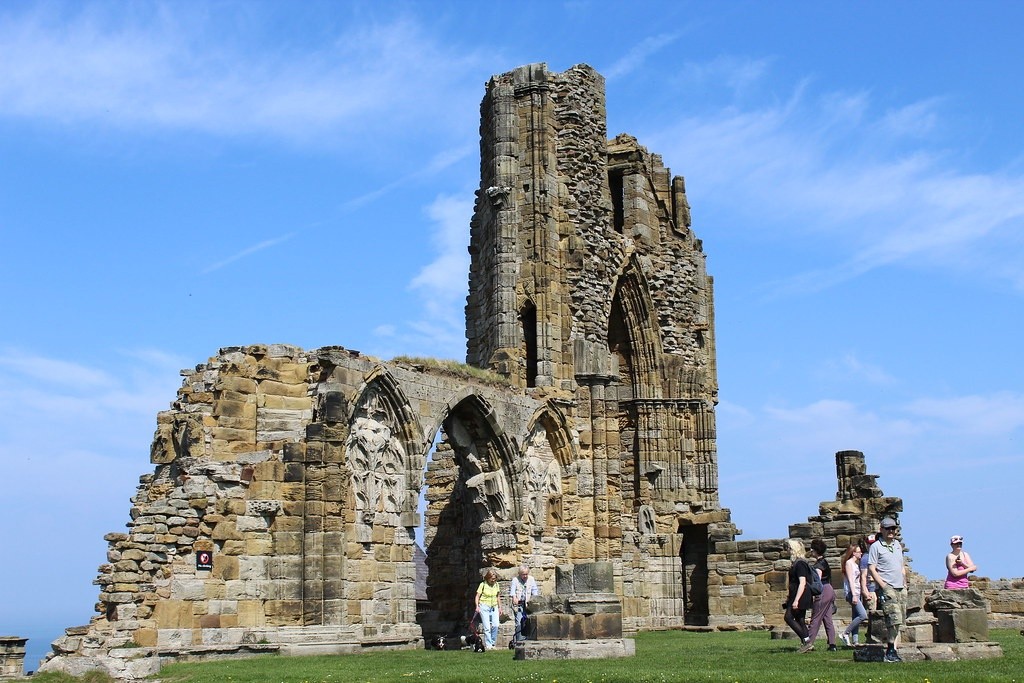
793, 558, 823, 595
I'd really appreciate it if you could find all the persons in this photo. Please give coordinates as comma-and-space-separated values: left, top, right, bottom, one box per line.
781, 540, 815, 654
474, 570, 505, 650
840, 544, 868, 648
867, 518, 907, 663
944, 536, 976, 590
875, 531, 883, 542
510, 565, 539, 642
804, 539, 839, 652
862, 535, 879, 644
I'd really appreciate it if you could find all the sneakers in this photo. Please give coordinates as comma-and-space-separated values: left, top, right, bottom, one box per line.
884, 649, 903, 662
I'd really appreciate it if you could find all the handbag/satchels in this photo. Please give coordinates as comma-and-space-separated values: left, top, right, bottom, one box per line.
831, 601, 837, 615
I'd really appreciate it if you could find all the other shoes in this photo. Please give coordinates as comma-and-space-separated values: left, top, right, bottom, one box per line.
797, 631, 881, 653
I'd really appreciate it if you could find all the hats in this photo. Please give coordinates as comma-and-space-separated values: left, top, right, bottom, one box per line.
880, 518, 898, 528
950, 535, 964, 544
865, 534, 876, 543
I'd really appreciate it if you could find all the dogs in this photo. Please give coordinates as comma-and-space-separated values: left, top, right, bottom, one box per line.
460, 635, 485, 653
431, 635, 449, 651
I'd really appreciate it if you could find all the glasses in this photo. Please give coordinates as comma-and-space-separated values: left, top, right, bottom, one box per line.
951, 536, 963, 540
786, 545, 791, 549
810, 547, 815, 552
881, 526, 897, 530
